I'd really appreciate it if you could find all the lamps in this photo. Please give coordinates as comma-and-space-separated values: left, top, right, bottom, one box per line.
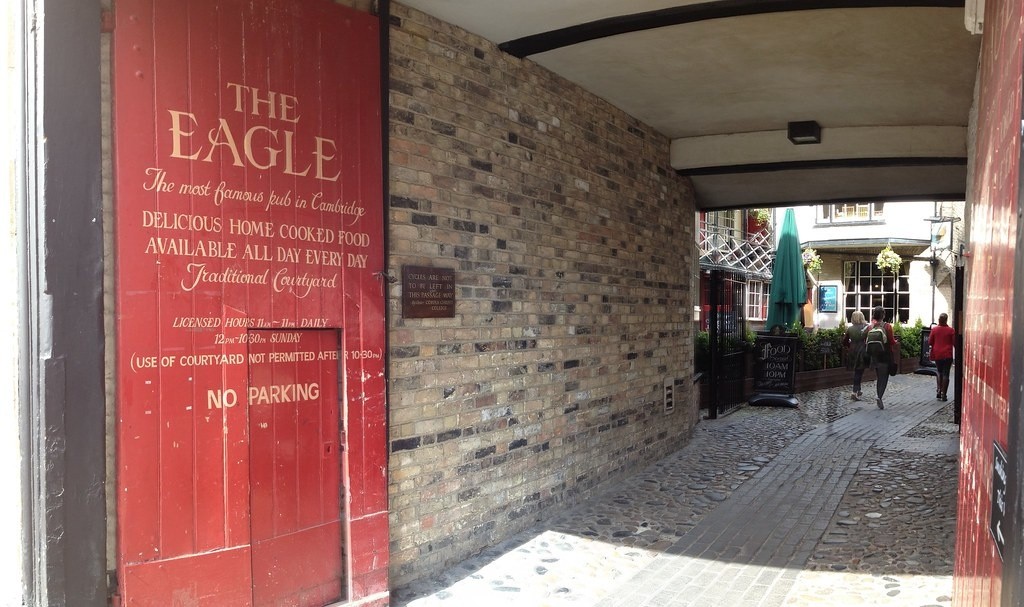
786, 121, 822, 146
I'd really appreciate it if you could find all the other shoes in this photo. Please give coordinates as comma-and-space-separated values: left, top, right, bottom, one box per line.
941, 394, 946, 400
876, 398, 883, 408
850, 394, 860, 400
937, 394, 941, 397
857, 392, 862, 396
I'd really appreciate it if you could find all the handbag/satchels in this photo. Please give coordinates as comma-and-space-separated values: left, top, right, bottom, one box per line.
889, 352, 898, 376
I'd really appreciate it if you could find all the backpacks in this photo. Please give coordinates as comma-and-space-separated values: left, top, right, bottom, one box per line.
866, 321, 889, 353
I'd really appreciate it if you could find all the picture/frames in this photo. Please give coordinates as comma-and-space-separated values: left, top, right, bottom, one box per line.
817, 284, 839, 313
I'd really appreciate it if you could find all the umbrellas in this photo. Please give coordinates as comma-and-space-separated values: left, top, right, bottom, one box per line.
765, 208, 808, 330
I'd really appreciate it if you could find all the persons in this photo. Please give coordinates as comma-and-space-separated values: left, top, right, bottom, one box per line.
843, 311, 871, 401
928, 313, 955, 401
864, 306, 898, 409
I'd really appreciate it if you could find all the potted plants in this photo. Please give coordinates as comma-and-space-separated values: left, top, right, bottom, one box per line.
750, 208, 771, 227
802, 247, 823, 288
875, 246, 903, 274
